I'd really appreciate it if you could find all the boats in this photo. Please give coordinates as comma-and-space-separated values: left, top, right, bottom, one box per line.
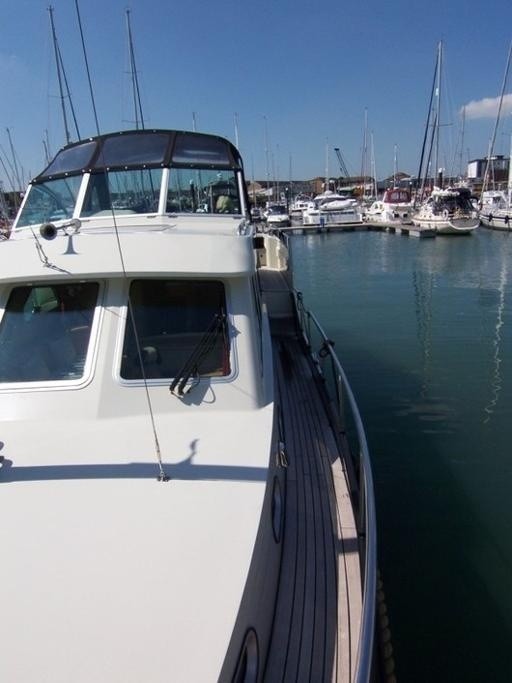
0, 130, 395, 682
